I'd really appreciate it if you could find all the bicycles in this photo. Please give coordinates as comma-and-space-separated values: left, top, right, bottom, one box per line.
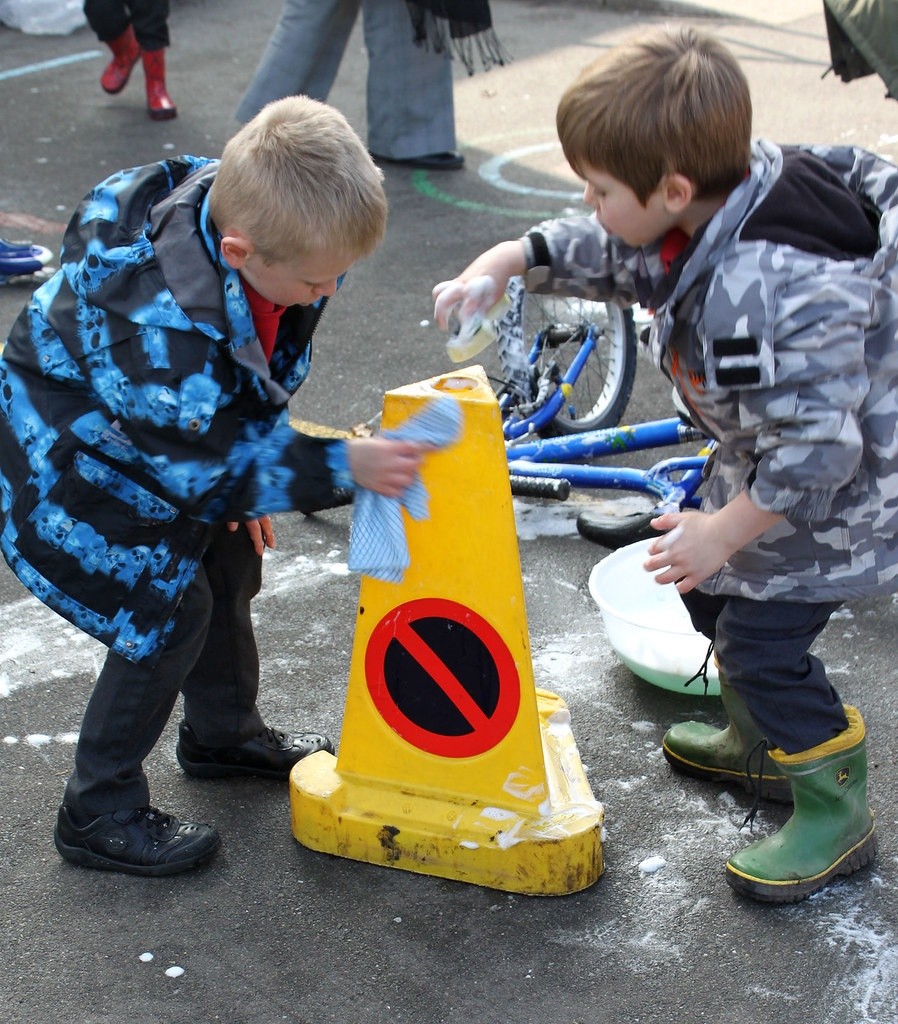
490, 269, 721, 553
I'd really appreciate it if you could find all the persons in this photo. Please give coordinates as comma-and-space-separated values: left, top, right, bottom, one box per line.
84, 0, 178, 119
0, 96, 431, 878
432, 30, 898, 902
236, 0, 466, 169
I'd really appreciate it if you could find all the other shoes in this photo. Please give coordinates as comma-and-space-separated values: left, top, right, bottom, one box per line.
173, 721, 331, 782
366, 146, 464, 170
52, 798, 222, 878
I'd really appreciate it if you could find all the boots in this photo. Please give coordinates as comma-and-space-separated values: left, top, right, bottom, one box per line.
141, 44, 178, 121
724, 702, 881, 905
99, 24, 140, 96
660, 657, 798, 806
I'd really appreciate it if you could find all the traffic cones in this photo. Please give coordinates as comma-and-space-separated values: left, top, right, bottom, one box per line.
286, 366, 604, 897
